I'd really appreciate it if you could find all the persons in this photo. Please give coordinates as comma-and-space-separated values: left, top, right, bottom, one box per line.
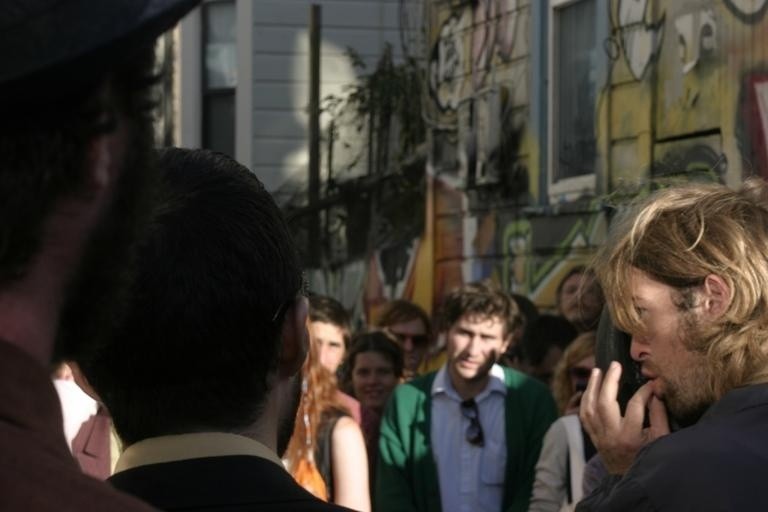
65, 146, 358, 512
279, 267, 642, 512
573, 173, 768, 511
1, 1, 162, 511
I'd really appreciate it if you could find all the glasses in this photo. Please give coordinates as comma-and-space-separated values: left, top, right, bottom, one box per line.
460, 398, 484, 448
